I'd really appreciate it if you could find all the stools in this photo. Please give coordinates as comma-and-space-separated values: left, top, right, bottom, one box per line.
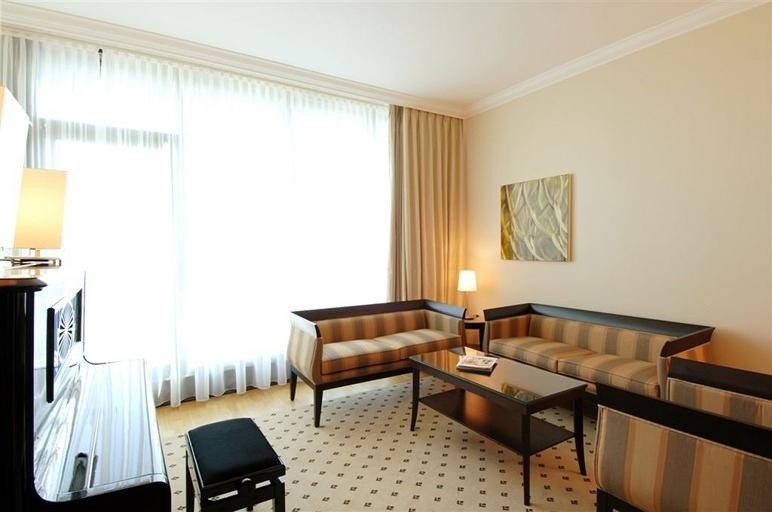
185, 417, 286, 512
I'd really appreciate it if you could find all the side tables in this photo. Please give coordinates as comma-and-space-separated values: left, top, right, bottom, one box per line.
465, 320, 485, 351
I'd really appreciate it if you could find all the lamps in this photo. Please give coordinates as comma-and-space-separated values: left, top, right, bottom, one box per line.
458, 269, 478, 319
11, 167, 67, 261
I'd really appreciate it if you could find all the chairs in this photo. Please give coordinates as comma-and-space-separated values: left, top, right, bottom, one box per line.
593, 354, 772, 512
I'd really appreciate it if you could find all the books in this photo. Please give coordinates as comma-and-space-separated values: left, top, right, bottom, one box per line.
457, 354, 497, 373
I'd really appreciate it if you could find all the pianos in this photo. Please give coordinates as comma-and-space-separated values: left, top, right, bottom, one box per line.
0, 260, 172, 512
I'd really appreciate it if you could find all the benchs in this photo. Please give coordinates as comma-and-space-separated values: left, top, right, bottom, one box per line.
285, 297, 468, 426
483, 303, 717, 420
